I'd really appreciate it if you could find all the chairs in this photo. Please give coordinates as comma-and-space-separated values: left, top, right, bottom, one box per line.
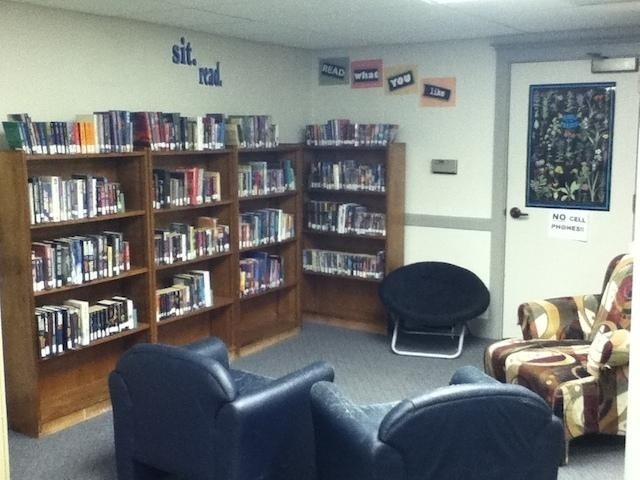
483, 252, 634, 465
309, 363, 569, 479
377, 257, 493, 360
105, 335, 336, 480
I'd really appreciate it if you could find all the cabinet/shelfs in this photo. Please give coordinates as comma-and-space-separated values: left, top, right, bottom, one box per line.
148, 133, 233, 362
233, 134, 306, 359
3, 145, 153, 438
299, 144, 406, 338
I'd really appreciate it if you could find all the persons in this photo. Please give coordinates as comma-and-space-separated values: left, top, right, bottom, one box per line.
550, 115, 583, 203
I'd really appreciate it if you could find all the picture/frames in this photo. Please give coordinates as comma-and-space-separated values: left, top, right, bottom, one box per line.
523, 80, 616, 213
